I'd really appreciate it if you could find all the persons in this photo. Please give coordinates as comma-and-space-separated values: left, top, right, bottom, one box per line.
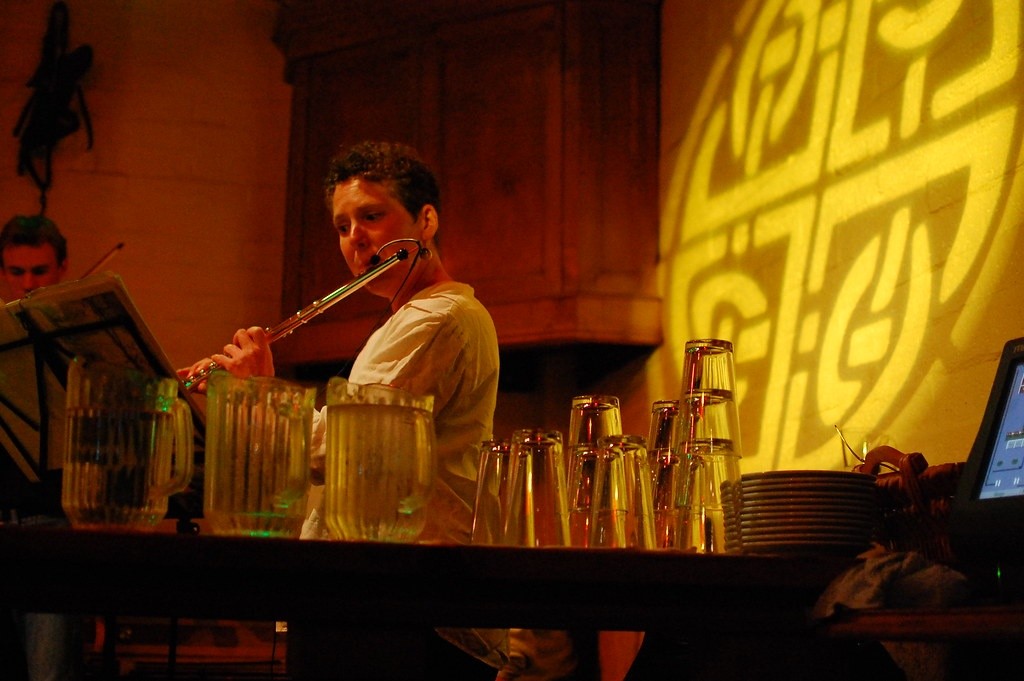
177, 143, 511, 681
0, 216, 69, 299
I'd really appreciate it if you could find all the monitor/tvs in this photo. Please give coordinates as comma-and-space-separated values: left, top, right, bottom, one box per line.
948, 336, 1024, 606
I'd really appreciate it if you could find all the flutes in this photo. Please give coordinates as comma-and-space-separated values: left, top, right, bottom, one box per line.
181, 246, 411, 391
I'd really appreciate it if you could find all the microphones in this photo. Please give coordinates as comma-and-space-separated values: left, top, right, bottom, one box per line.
369, 254, 381, 266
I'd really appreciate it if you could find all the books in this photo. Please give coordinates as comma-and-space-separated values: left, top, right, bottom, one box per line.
0, 271, 206, 481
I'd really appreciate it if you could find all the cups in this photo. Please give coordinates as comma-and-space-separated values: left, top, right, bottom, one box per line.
471, 338, 742, 555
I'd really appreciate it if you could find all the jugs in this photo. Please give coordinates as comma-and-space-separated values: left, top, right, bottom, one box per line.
326, 377, 437, 542
60, 355, 194, 530
205, 370, 316, 537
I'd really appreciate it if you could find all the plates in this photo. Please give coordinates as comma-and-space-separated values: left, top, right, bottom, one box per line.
720, 470, 878, 552
